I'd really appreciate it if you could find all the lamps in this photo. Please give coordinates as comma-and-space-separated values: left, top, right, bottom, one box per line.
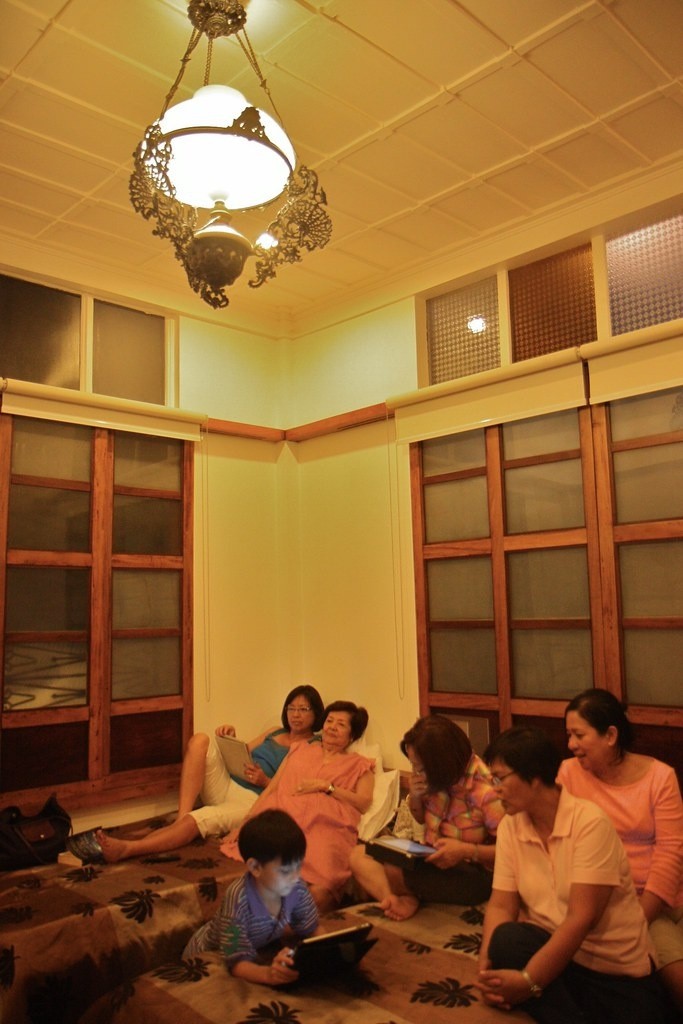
128, 0, 335, 310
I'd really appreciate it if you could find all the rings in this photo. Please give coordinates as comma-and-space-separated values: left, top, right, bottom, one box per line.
298, 786, 301, 791
249, 774, 253, 779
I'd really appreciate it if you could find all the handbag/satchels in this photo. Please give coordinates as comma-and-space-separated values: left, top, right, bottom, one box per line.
0, 792, 73, 872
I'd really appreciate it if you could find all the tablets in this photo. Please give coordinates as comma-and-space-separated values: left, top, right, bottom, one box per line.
370, 837, 437, 859
216, 734, 254, 780
285, 922, 372, 971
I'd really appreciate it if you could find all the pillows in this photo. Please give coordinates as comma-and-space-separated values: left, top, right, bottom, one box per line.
356, 745, 401, 842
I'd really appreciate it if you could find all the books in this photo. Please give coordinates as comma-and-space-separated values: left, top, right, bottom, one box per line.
57, 826, 105, 867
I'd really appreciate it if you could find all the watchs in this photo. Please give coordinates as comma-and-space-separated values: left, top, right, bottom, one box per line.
464, 843, 478, 864
521, 968, 543, 998
326, 782, 335, 795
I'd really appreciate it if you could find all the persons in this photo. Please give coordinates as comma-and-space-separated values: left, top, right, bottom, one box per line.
473, 726, 660, 1024
169, 808, 330, 986
221, 700, 375, 921
552, 689, 683, 995
96, 685, 326, 863
348, 714, 507, 920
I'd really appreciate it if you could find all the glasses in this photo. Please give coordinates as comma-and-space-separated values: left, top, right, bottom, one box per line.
415, 766, 425, 777
287, 705, 310, 713
491, 771, 513, 787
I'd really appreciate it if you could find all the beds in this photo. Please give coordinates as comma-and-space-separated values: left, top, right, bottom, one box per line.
0, 768, 541, 1024
